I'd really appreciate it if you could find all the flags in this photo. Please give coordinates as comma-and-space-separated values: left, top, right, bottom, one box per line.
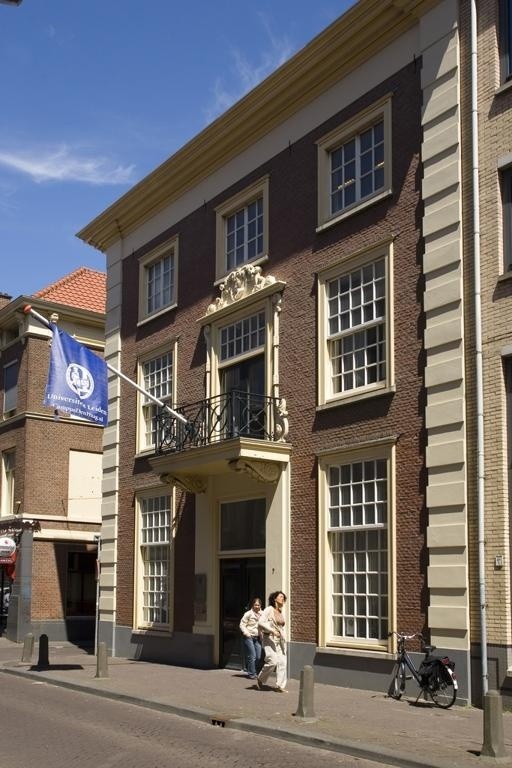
42, 322, 109, 426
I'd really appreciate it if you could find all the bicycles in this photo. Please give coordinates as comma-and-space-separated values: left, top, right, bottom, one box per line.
379, 626, 460, 710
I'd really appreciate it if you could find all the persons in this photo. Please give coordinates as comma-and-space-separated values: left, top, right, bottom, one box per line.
238, 596, 264, 680
258, 591, 288, 692
